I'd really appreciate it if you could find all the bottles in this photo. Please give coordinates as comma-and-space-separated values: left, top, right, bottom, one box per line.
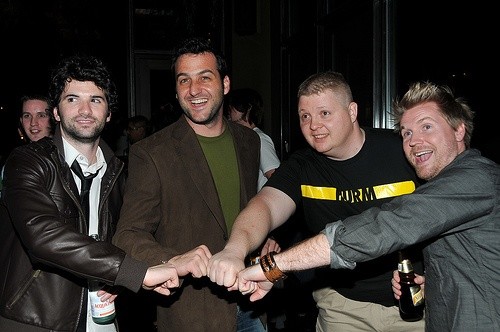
397, 249, 424, 322
247, 240, 261, 267
88, 234, 116, 324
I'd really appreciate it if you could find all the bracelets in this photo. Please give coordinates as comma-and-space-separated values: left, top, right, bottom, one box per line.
259, 251, 288, 285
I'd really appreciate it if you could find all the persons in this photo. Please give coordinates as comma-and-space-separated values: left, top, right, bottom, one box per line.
237, 83, 500, 332
113, 37, 268, 332
125, 115, 150, 143
206, 72, 425, 332
228, 87, 280, 191
20, 92, 52, 142
0, 54, 180, 332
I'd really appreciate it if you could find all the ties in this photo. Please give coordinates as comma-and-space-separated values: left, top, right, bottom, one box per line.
70, 158, 101, 237
